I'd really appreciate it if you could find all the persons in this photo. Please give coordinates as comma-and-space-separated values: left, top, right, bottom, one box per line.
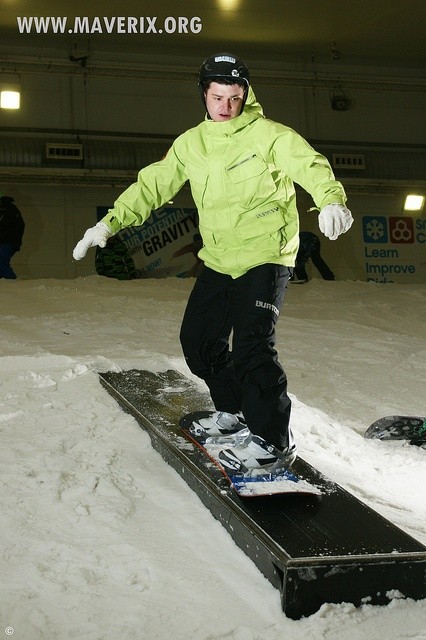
170, 234, 203, 277
72, 54, 354, 470
0, 196, 24, 279
288, 232, 335, 284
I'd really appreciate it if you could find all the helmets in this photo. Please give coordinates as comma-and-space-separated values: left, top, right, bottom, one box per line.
197, 53, 249, 88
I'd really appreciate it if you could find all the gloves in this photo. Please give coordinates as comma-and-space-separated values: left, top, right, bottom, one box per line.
318, 204, 354, 241
72, 221, 113, 260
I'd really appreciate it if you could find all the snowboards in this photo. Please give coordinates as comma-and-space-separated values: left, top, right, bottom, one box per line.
179, 410, 322, 496
364, 415, 426, 440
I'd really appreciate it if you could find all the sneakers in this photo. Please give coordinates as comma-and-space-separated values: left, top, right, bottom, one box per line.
219, 432, 297, 471
188, 411, 240, 437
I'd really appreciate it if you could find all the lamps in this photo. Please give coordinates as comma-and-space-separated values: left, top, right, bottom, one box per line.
330, 89, 351, 111
